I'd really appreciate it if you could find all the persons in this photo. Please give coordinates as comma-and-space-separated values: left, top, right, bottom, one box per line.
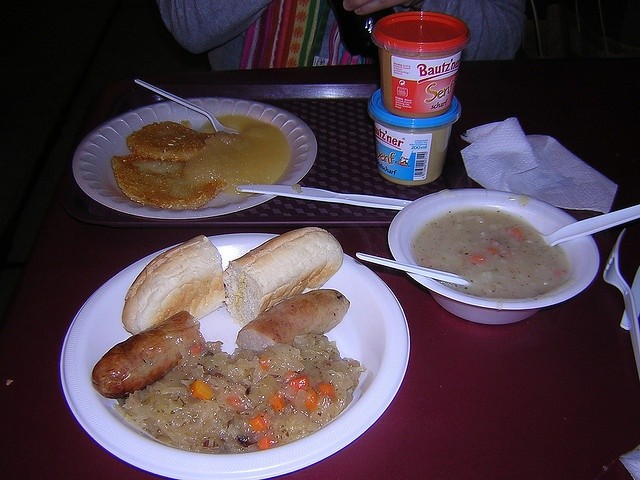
154, 0, 528, 71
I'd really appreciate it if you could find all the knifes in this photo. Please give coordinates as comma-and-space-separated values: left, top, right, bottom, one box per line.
233, 182, 415, 213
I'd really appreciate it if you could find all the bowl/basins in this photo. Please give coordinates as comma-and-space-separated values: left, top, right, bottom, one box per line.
385, 189, 601, 328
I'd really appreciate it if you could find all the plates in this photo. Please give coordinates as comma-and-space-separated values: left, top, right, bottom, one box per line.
70, 96, 318, 221
57, 231, 409, 475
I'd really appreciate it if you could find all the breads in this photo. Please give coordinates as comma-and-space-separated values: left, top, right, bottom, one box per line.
121, 235, 226, 337
223, 227, 343, 328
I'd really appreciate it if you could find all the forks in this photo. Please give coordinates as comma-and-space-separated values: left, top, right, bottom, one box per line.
601, 228, 640, 379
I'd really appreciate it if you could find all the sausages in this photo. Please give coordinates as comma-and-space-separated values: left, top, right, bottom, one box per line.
235, 289, 350, 348
92, 310, 204, 399
